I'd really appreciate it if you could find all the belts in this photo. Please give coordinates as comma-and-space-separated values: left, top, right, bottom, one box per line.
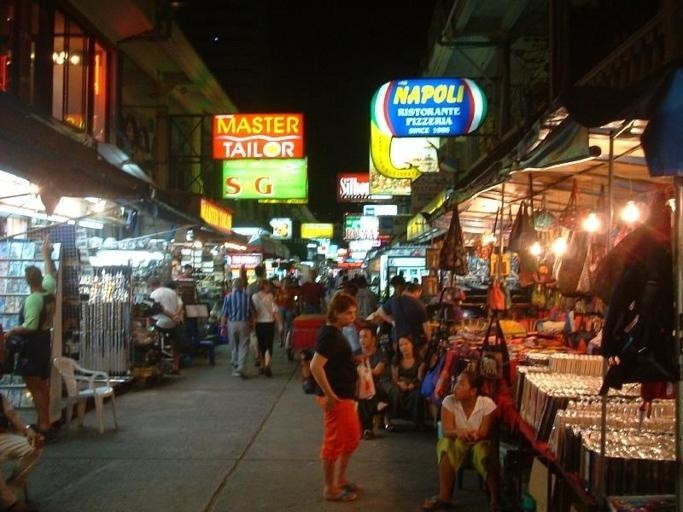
82, 265, 127, 358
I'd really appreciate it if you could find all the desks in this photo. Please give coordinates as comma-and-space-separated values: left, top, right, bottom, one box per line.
478, 379, 609, 507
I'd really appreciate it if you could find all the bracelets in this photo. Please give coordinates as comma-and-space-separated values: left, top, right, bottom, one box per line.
22, 425, 34, 435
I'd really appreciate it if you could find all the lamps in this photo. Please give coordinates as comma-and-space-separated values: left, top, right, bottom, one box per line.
525, 127, 643, 257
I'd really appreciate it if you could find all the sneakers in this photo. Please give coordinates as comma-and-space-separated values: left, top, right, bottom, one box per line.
254, 357, 272, 376
234, 371, 249, 380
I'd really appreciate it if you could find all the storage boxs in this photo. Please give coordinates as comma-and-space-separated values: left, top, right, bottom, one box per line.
427, 405, 439, 427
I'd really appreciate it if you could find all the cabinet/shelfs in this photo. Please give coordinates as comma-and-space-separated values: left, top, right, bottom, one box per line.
134, 259, 231, 389
79, 265, 133, 375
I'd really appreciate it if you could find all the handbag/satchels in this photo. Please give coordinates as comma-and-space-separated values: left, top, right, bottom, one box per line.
551, 251, 599, 297
421, 275, 438, 296
559, 206, 585, 233
417, 349, 458, 406
425, 248, 440, 271
508, 215, 538, 252
530, 284, 546, 309
490, 238, 511, 276
489, 283, 512, 311
439, 231, 468, 276
466, 343, 509, 383
533, 209, 558, 232
300, 348, 325, 397
2, 332, 30, 385
436, 287, 463, 325
589, 195, 680, 397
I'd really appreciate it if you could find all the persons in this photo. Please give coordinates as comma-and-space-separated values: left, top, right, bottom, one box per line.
0, 382, 43, 511
303, 292, 363, 503
417, 368, 507, 510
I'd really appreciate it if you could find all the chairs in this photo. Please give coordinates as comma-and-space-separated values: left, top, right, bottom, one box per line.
436, 420, 484, 502
53, 353, 119, 439
1, 430, 45, 507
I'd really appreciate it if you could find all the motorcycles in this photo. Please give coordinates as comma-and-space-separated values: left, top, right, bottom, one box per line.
283, 295, 329, 364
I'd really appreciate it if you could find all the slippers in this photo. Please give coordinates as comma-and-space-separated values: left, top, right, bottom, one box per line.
322, 486, 356, 502
422, 494, 454, 511
336, 478, 359, 491
